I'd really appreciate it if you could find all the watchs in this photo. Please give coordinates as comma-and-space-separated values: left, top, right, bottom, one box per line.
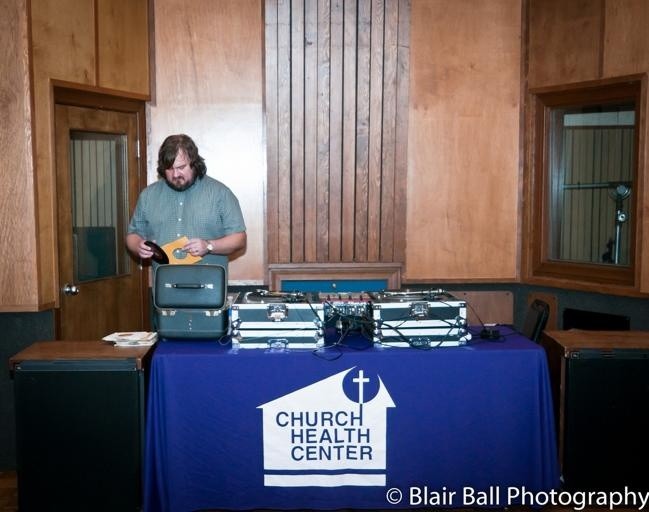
206, 241, 214, 257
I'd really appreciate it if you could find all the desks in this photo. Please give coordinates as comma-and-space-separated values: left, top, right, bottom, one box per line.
143, 321, 562, 512
541, 328, 649, 478
8, 339, 159, 512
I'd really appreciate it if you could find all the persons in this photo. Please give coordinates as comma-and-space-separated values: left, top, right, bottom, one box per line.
124, 135, 246, 296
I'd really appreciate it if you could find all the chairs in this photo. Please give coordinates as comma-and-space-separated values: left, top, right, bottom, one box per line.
561, 307, 631, 331
520, 299, 551, 344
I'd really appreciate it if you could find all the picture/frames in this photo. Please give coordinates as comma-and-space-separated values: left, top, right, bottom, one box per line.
268, 261, 402, 289
